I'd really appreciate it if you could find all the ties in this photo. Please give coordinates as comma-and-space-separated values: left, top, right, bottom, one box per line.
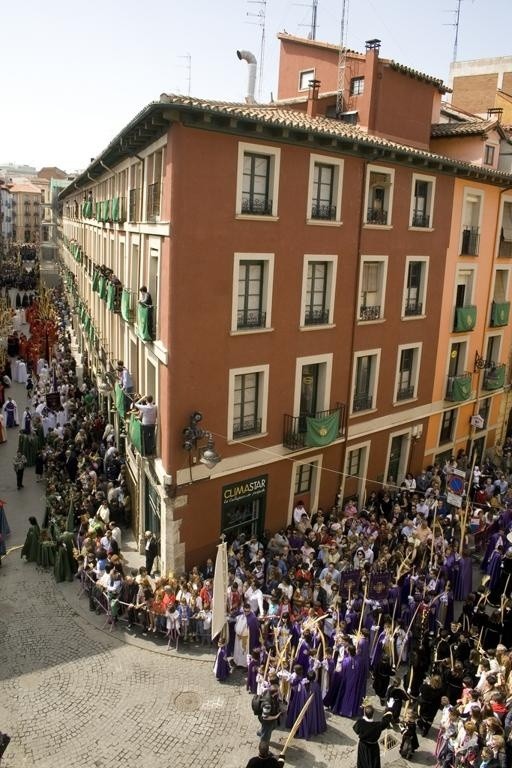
209, 568, 210, 573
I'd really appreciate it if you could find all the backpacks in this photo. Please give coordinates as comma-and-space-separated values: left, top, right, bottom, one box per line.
252, 691, 267, 715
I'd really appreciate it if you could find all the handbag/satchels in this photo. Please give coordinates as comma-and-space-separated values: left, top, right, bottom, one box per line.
296, 596, 305, 607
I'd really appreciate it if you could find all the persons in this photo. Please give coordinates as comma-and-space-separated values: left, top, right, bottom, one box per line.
60, 192, 154, 342
150, 444, 510, 768
0, 266, 156, 615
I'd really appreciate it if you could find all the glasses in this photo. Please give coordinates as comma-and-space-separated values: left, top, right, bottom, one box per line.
332, 589, 337, 591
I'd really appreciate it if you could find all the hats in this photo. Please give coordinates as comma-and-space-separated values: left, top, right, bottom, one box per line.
496, 643, 508, 651
164, 585, 172, 593
104, 530, 112, 537
